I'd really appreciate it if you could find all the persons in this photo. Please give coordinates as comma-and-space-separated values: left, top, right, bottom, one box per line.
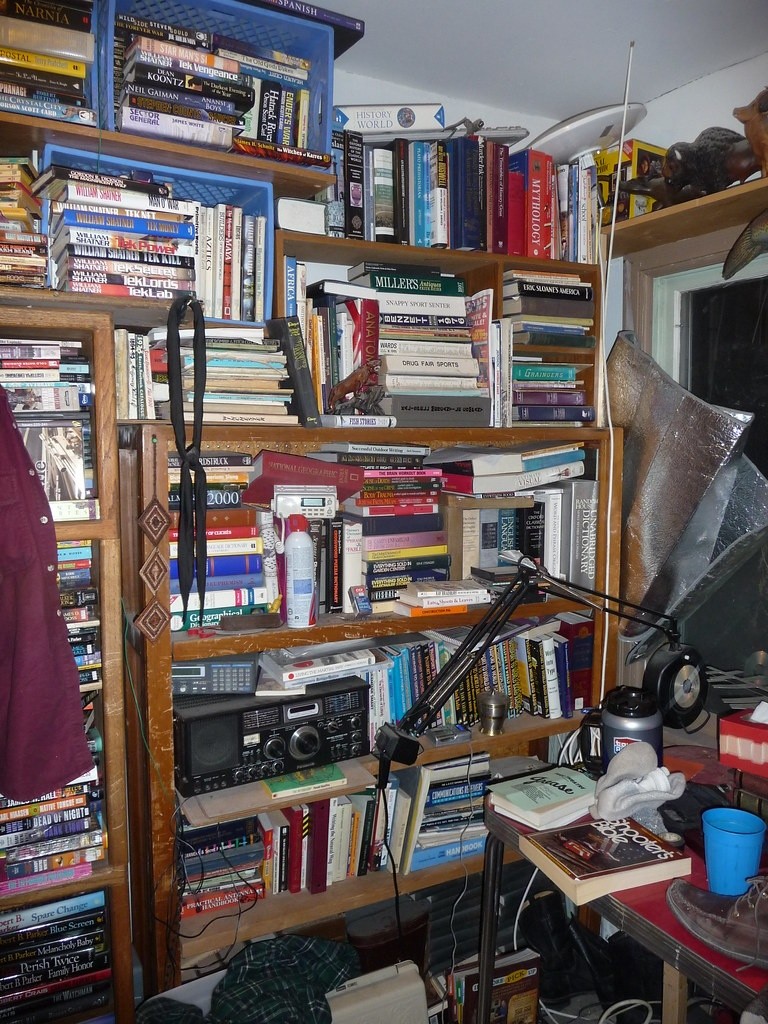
634, 156, 651, 176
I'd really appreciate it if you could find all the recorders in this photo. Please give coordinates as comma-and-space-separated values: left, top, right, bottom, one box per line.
174, 676, 371, 798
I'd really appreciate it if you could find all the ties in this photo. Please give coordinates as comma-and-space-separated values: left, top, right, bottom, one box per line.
168, 294, 207, 626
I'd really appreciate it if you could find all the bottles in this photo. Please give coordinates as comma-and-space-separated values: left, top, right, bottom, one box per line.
285, 514, 317, 628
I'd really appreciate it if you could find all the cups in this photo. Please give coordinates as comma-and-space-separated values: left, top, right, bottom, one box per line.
702, 808, 766, 896
601, 685, 662, 774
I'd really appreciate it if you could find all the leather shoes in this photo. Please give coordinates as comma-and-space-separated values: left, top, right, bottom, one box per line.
667, 874, 767, 972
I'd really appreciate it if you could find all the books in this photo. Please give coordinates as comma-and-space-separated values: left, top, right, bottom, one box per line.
0, 0, 768, 1024
520, 816, 691, 906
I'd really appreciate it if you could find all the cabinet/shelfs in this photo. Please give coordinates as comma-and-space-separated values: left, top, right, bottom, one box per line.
0, 0, 768, 1024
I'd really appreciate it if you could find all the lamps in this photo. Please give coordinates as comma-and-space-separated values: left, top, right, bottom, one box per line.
372, 556, 710, 790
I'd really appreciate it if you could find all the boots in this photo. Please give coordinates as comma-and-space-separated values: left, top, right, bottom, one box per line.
568, 912, 664, 1024
519, 889, 607, 1011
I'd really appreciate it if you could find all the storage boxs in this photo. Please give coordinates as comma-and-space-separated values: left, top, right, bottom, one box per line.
84, 0, 99, 127
592, 139, 668, 227
99, 0, 333, 174
40, 141, 275, 329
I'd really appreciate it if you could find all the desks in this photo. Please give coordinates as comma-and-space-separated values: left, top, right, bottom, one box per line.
476, 794, 768, 1024
